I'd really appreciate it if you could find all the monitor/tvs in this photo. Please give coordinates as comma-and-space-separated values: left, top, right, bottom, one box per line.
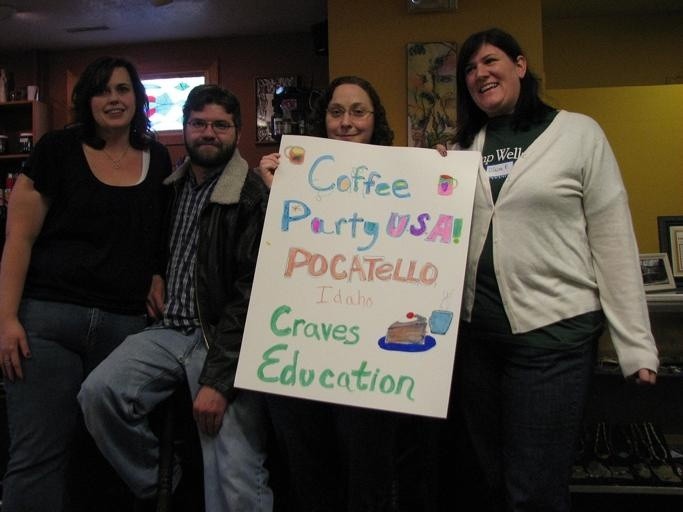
140, 76, 205, 131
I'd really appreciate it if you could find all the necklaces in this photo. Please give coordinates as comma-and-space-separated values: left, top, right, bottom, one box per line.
98, 144, 130, 171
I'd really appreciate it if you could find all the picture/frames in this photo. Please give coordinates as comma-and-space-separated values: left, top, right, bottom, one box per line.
252, 74, 301, 146
656, 217, 682, 281
639, 253, 678, 291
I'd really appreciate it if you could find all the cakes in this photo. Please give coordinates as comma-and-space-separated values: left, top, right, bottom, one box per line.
385, 312, 427, 347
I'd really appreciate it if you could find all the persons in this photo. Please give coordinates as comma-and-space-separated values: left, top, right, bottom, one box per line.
254, 75, 423, 511
75, 82, 276, 511
427, 28, 660, 512
0, 54, 176, 511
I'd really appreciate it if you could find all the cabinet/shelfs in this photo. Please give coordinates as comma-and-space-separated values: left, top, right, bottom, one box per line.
0, 100, 50, 214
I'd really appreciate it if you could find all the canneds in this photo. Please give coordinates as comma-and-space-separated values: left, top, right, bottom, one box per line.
1, 135, 8, 155
19, 132, 33, 153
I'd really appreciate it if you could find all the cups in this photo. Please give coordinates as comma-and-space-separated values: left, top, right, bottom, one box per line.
27, 85, 38, 102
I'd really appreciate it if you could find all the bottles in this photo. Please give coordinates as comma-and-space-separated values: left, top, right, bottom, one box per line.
0, 172, 13, 208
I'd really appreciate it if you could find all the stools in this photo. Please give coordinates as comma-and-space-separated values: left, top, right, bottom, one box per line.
149, 388, 196, 510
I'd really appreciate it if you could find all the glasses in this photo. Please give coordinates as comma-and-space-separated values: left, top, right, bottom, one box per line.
184, 118, 237, 133
325, 105, 376, 119
